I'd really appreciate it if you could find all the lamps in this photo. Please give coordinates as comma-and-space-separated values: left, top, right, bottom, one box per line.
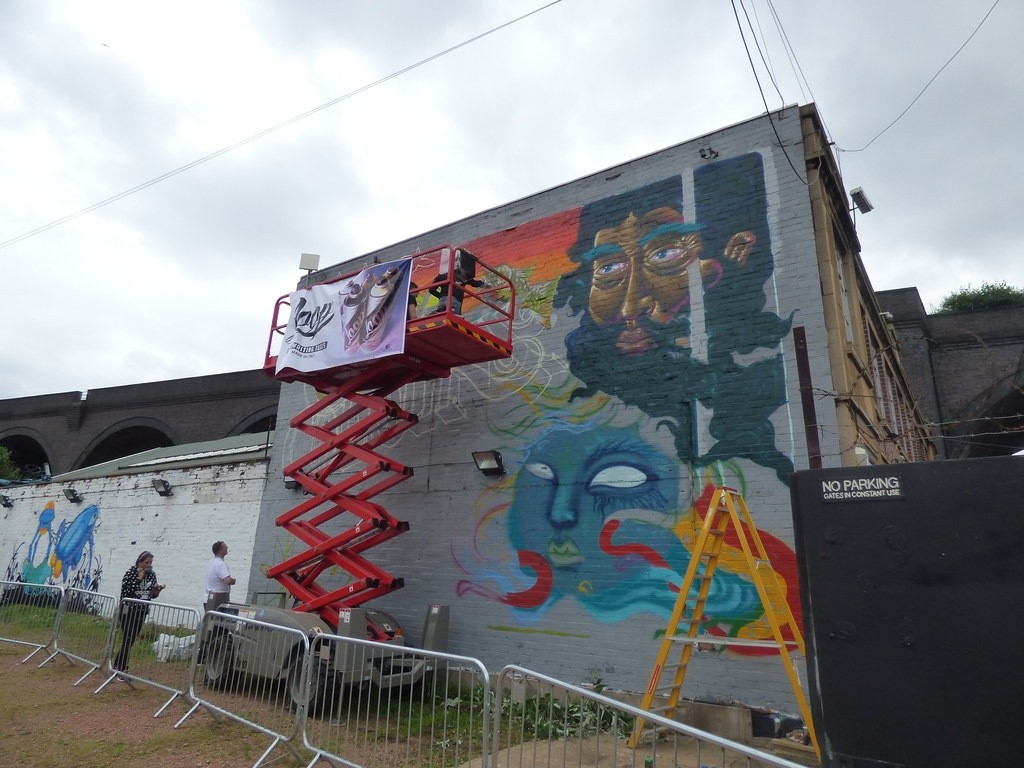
0, 495, 13, 508
699, 145, 719, 160
62, 489, 84, 503
470, 450, 507, 477
879, 311, 894, 321
152, 479, 174, 497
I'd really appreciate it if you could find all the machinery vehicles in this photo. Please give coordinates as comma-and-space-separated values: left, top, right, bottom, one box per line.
206, 245, 518, 715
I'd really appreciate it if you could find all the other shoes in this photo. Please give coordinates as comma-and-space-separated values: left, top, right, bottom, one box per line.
111, 665, 131, 682
197, 660, 207, 667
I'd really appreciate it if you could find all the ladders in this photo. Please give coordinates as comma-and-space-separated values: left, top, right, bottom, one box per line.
627, 484, 822, 765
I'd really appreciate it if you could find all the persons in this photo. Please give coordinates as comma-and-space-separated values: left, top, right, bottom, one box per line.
112, 550, 167, 682
196, 540, 237, 668
